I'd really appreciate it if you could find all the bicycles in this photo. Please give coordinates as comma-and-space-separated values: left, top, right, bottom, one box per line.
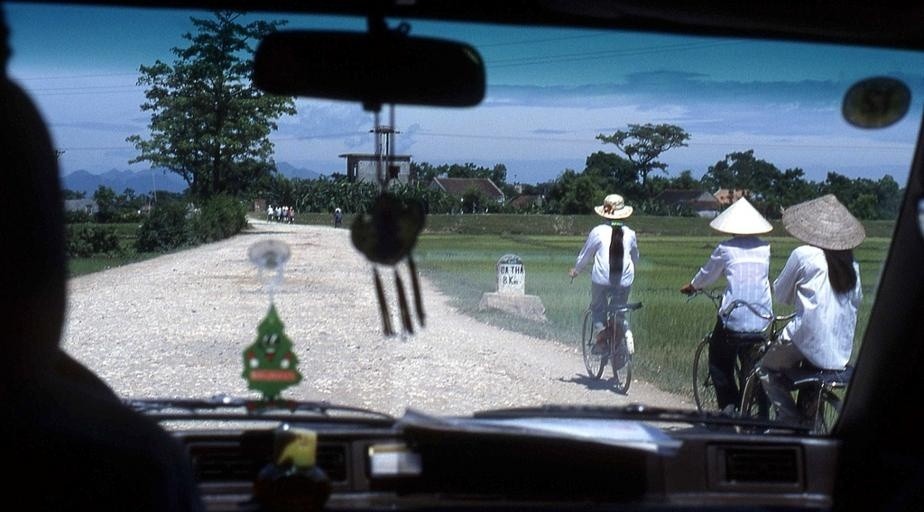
683, 285, 855, 436
567, 268, 643, 394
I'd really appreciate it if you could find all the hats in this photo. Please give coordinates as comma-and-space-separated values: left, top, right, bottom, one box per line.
594, 194, 633, 219
709, 196, 773, 234
782, 193, 866, 251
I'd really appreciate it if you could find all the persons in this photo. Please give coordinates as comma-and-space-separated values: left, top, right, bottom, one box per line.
569, 194, 640, 355
266, 204, 295, 224
333, 205, 343, 229
680, 196, 774, 420
754, 194, 865, 424
